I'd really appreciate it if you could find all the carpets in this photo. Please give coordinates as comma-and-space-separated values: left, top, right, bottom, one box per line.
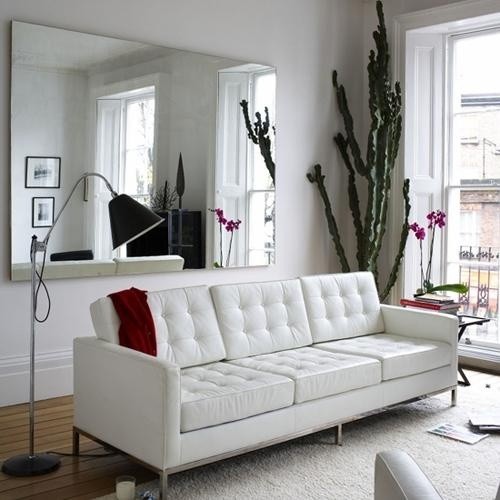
93, 397, 499, 500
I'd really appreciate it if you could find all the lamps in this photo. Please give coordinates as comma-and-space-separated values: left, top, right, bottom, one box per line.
0, 173, 165, 477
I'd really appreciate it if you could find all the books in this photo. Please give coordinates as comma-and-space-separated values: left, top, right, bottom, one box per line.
469, 417, 500, 427
400, 292, 463, 322
426, 422, 492, 445
478, 426, 500, 430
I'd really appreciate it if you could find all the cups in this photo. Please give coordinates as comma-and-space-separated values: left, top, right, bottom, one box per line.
115, 475, 137, 500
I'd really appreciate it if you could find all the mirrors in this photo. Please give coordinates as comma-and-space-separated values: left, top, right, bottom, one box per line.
8, 19, 278, 283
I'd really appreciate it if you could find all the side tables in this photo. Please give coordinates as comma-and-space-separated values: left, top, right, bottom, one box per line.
458, 315, 490, 386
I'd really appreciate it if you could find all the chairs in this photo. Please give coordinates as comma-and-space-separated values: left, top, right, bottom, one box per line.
374, 449, 443, 500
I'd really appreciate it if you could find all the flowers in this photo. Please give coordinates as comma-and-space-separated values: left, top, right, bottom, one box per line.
408, 209, 468, 295
208, 208, 243, 266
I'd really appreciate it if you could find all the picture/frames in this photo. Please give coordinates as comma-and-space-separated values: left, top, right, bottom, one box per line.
25, 156, 61, 188
33, 197, 55, 228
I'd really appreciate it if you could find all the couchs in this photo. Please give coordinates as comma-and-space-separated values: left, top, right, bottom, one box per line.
11, 254, 184, 281
72, 271, 458, 500
